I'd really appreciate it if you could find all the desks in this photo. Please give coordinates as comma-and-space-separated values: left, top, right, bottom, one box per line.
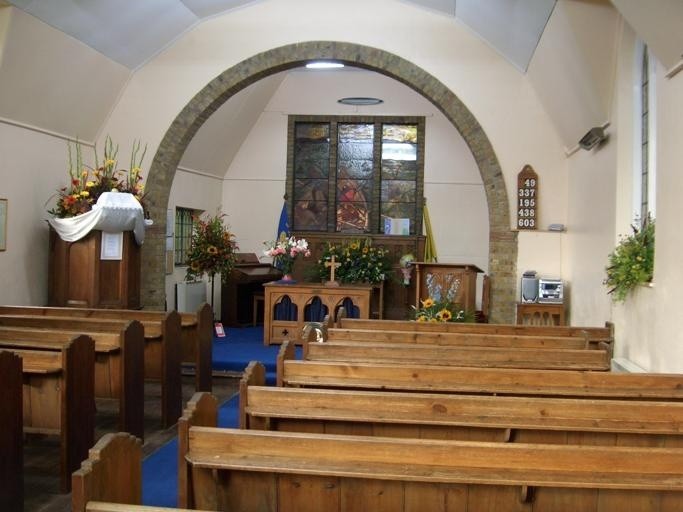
263, 279, 373, 346
517, 302, 566, 326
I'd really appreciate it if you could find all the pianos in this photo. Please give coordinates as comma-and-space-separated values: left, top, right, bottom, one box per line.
221, 253, 285, 328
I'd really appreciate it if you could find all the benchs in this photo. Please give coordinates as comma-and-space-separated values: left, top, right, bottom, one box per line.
1, 303, 215, 510
72, 307, 683, 511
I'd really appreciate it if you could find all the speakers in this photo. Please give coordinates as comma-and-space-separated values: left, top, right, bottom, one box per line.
521, 278, 539, 304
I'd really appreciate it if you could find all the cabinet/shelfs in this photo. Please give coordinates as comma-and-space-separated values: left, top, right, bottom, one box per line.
50, 226, 140, 312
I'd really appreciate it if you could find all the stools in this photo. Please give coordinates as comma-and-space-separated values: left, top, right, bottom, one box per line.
252, 290, 265, 326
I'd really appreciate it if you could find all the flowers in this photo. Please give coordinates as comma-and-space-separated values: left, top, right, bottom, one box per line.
46, 132, 151, 220
260, 230, 415, 285
183, 206, 238, 284
411, 271, 479, 326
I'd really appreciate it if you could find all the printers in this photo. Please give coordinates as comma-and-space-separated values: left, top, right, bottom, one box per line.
539, 277, 563, 305
